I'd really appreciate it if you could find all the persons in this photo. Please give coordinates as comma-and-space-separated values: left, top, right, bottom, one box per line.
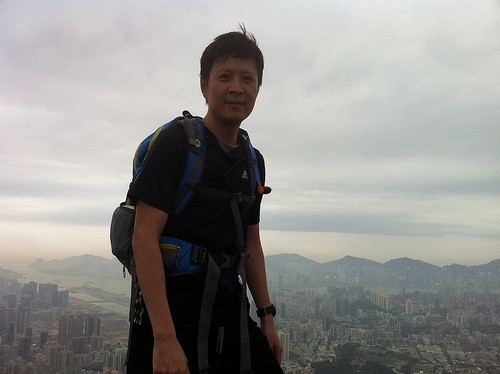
125, 31, 286, 374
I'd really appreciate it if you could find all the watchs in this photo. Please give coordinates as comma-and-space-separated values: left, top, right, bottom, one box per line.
256, 303, 276, 318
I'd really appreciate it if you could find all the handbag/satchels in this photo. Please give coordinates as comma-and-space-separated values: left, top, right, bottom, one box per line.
157, 235, 220, 278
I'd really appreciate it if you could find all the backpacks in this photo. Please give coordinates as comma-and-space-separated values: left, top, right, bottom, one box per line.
110, 110, 275, 278
